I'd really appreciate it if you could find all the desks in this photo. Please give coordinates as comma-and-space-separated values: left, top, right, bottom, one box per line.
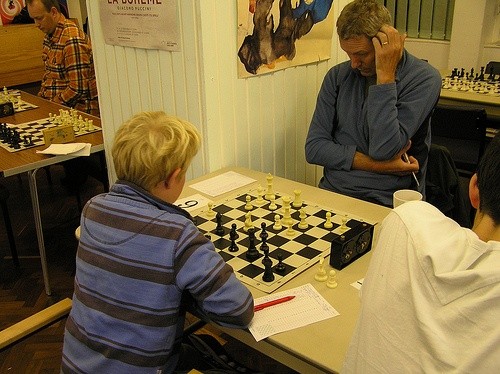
438, 75, 500, 136
74, 165, 396, 374
0, 88, 105, 295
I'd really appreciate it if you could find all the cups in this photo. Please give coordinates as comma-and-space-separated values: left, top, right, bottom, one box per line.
393, 189, 423, 208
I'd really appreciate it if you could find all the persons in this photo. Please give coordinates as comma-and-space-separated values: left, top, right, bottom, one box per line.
339, 132, 500, 374
304, 0, 442, 209
26, 0, 99, 118
61, 109, 255, 374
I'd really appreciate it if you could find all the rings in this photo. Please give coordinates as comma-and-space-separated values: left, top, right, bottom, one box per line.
382, 41, 388, 46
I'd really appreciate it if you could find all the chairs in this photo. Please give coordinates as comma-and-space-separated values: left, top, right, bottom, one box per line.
425, 108, 488, 228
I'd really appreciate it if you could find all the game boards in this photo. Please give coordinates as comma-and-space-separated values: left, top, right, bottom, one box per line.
0, 115, 102, 152
441, 74, 500, 97
0, 92, 39, 113
191, 185, 379, 293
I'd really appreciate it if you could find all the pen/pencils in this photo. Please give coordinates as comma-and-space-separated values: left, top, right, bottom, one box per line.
403, 152, 420, 186
254, 295, 297, 313
